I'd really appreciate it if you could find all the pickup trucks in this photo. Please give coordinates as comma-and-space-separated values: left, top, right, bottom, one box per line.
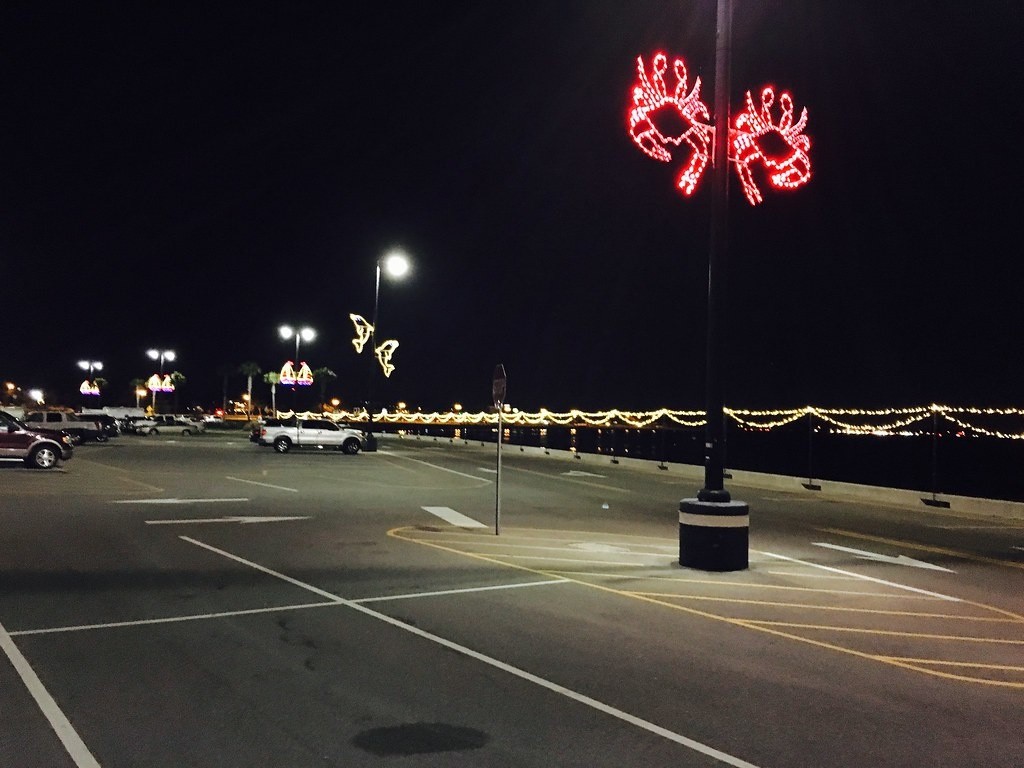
249, 419, 368, 455
18, 410, 102, 446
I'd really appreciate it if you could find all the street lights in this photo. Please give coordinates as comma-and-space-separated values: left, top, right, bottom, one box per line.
148, 349, 175, 413
78, 361, 103, 409
279, 326, 315, 419
21, 389, 44, 410
363, 251, 409, 452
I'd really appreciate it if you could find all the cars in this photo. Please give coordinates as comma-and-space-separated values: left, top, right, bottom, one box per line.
137, 420, 198, 436
78, 414, 223, 435
0, 411, 74, 469
